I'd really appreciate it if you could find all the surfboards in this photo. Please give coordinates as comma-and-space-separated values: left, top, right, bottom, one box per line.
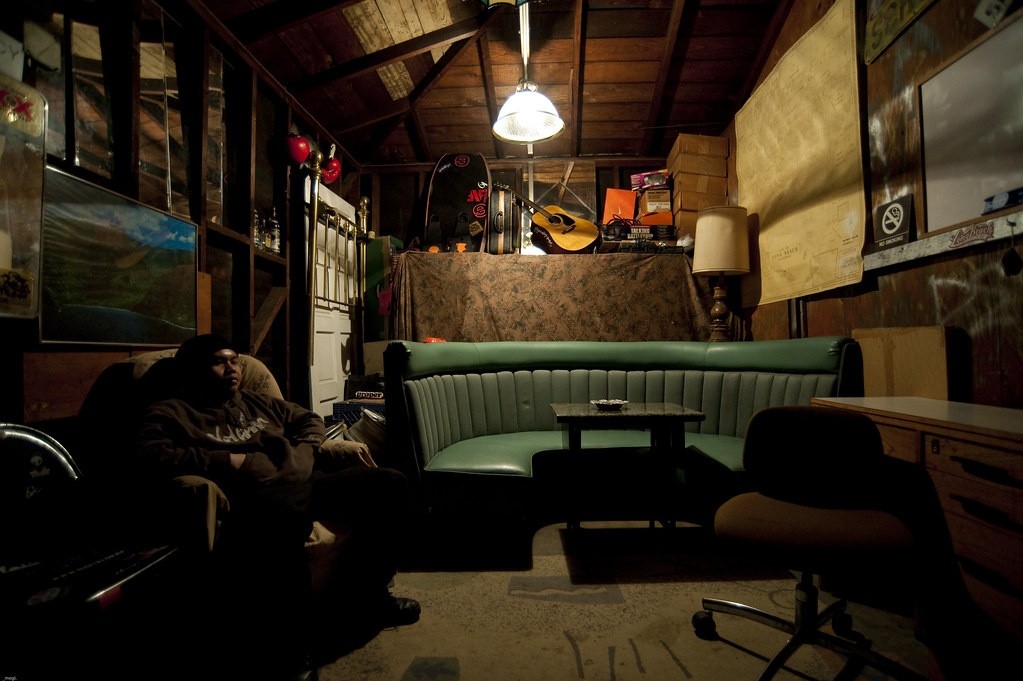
423, 152, 493, 253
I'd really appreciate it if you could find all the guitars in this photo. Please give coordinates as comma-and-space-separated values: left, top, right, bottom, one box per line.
495, 181, 601, 254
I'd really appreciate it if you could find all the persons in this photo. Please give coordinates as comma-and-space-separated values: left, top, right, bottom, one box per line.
132, 335, 421, 681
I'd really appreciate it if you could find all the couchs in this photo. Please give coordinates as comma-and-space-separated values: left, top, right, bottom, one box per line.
101, 349, 378, 604
384, 335, 864, 521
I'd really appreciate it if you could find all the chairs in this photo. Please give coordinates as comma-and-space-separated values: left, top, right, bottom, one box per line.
693, 406, 925, 681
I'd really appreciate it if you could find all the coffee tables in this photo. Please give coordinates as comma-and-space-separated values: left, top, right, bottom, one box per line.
549, 402, 702, 537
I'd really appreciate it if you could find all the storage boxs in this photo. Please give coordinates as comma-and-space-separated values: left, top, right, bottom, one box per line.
667, 133, 728, 170
675, 211, 697, 243
602, 187, 636, 225
672, 191, 728, 214
634, 212, 673, 226
668, 154, 727, 179
673, 172, 728, 199
334, 399, 386, 428
638, 190, 670, 216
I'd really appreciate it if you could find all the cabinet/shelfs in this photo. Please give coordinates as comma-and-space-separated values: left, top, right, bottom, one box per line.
304, 152, 370, 420
812, 396, 1023, 645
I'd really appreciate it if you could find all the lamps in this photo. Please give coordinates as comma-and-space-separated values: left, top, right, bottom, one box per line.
491, 0, 565, 145
692, 206, 750, 341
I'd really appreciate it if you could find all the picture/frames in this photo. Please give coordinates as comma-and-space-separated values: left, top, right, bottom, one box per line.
864, 0, 935, 65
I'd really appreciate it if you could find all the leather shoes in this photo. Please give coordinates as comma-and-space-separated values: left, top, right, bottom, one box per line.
346, 586, 420, 624
290, 642, 319, 681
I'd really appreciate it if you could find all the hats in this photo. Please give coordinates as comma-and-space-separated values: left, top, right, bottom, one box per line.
174, 334, 239, 375
